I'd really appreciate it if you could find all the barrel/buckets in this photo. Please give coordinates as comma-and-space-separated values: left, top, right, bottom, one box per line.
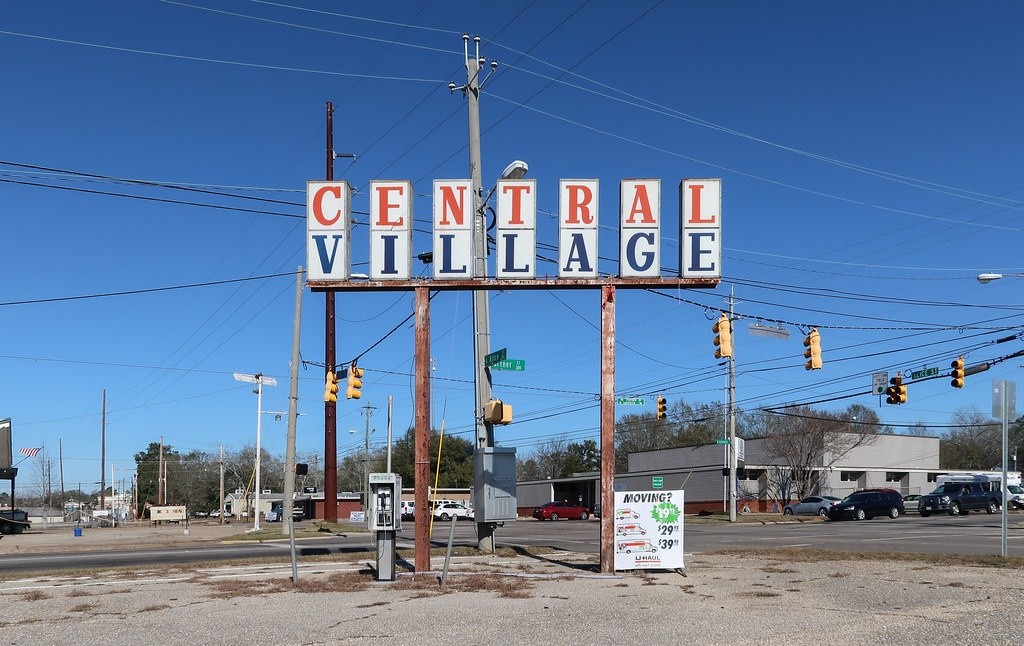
74, 528, 81, 536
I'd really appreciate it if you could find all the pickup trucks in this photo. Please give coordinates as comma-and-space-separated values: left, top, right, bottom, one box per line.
919, 484, 1002, 517
210, 510, 232, 518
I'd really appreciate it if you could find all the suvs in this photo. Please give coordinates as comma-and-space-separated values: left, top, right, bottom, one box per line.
401, 500, 415, 520
195, 509, 208, 518
270, 503, 305, 522
1007, 485, 1024, 510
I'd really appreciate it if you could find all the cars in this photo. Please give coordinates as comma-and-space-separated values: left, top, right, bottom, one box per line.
428, 500, 475, 521
842, 488, 924, 514
827, 492, 904, 521
91, 515, 118, 528
532, 502, 590, 521
783, 496, 844, 516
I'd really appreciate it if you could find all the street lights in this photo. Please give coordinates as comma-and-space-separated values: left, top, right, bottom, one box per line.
468, 160, 529, 555
803, 331, 822, 371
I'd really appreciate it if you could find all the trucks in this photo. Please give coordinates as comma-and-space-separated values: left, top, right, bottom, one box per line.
936, 475, 1002, 512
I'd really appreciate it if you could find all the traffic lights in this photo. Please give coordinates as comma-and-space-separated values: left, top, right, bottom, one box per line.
949, 356, 965, 389
347, 367, 364, 399
895, 385, 907, 403
890, 377, 902, 385
711, 316, 733, 358
886, 386, 896, 404
324, 371, 340, 401
657, 397, 667, 419
